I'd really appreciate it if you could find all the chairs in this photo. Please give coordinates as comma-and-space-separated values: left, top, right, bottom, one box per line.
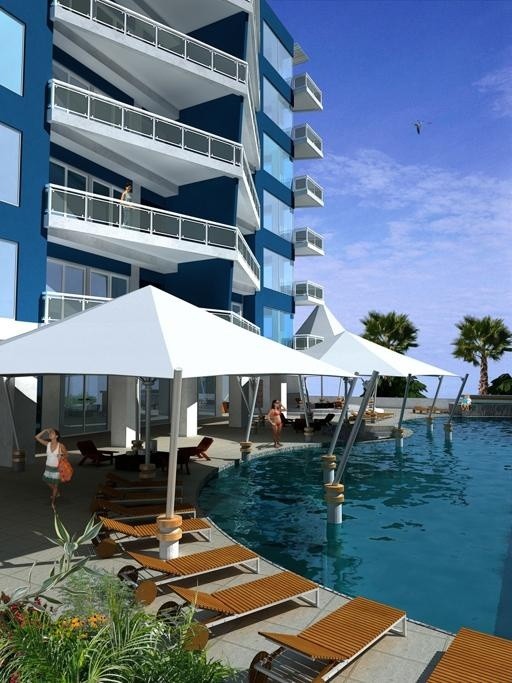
73, 433, 215, 559
115, 541, 260, 607
154, 570, 323, 654
249, 397, 431, 434
424, 627, 512, 683
249, 597, 408, 683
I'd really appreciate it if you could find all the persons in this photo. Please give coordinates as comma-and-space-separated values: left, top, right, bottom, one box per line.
266, 398, 288, 449
120, 180, 134, 227
459, 393, 472, 415
34, 427, 69, 517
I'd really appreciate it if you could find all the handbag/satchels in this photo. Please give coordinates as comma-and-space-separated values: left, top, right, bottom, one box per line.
59, 457, 74, 482
